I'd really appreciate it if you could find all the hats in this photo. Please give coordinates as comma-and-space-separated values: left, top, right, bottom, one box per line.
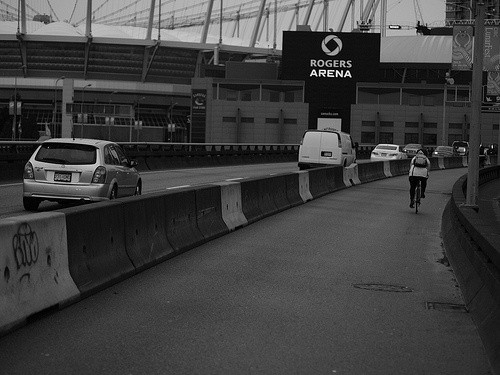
416, 148, 427, 153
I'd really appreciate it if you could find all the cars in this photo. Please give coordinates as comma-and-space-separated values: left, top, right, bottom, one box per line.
23, 137, 143, 210
370, 143, 409, 161
402, 143, 426, 158
432, 146, 460, 157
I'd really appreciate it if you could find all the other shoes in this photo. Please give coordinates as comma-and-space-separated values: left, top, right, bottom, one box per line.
421, 192, 426, 198
410, 201, 414, 207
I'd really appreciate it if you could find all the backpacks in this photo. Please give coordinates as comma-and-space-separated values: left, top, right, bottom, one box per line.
414, 153, 428, 168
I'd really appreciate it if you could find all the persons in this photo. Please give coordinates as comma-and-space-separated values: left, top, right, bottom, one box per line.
408, 147, 431, 208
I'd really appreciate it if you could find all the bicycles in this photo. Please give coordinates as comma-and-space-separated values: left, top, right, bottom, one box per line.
407, 170, 429, 215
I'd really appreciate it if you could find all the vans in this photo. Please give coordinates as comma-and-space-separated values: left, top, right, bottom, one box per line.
297, 128, 359, 170
452, 140, 469, 157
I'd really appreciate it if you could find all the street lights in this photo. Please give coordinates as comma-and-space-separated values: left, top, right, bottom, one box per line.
109, 90, 118, 141
14, 63, 26, 141
169, 101, 178, 142
53, 75, 66, 138
81, 84, 92, 138
137, 96, 147, 142
